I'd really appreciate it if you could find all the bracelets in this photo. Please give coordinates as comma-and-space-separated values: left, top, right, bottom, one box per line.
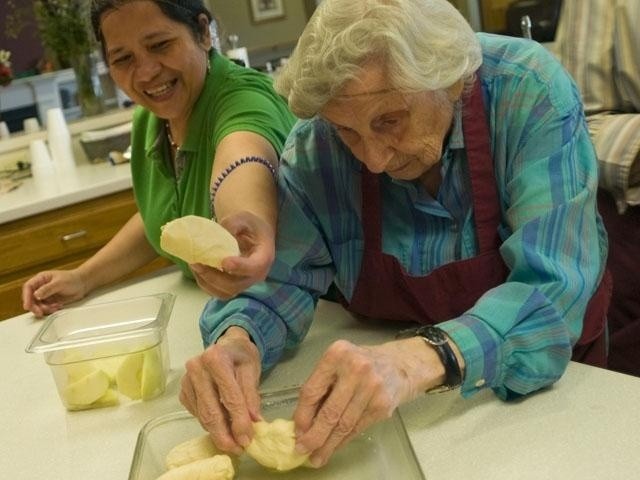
209, 154, 278, 219
398, 323, 464, 397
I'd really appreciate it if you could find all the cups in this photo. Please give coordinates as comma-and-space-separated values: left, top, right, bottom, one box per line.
29, 109, 77, 178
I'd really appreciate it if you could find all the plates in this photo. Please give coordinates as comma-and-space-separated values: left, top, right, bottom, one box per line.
126, 384, 425, 480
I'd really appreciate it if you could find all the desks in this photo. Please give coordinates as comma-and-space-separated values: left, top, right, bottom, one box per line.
0, 269, 639, 480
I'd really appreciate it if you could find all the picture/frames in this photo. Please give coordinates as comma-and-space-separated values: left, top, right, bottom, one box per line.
248, 0, 285, 25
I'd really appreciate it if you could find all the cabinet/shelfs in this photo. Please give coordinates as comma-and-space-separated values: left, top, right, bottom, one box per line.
0, 189, 173, 322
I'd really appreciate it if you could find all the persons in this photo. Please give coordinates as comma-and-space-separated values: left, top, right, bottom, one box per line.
552, 1, 640, 379
176, 0, 614, 469
20, 0, 304, 320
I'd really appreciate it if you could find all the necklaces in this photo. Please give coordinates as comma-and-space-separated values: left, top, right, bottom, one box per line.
163, 119, 182, 152
